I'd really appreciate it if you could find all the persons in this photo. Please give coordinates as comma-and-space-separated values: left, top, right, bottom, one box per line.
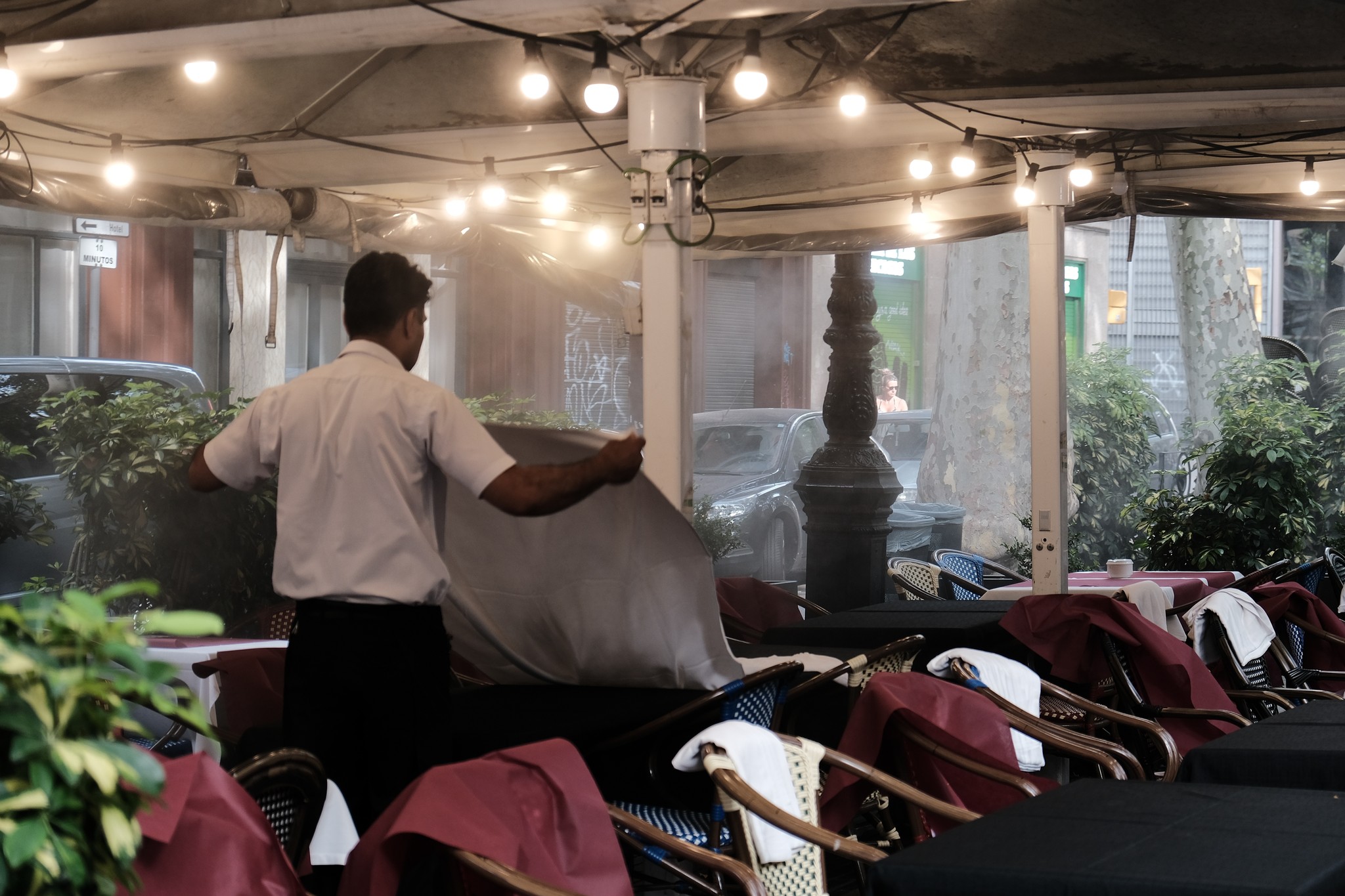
188, 248, 646, 862
873, 367, 909, 436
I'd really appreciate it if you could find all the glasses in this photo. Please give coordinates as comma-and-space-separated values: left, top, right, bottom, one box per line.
886, 386, 899, 390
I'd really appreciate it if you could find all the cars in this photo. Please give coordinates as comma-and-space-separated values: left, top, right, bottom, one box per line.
1121, 389, 1190, 508
692, 408, 933, 582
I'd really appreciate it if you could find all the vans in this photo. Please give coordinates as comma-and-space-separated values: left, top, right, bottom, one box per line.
0, 358, 223, 596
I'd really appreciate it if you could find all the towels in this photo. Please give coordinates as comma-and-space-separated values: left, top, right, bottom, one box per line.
303, 773, 364, 866
1182, 587, 1279, 665
1110, 580, 1187, 642
924, 645, 1046, 773
728, 651, 850, 686
669, 716, 815, 870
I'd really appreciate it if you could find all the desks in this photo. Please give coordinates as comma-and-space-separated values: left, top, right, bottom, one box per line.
839, 778, 1345, 896
764, 596, 1030, 664
980, 571, 1243, 640
446, 683, 716, 788
1177, 700, 1345, 791
126, 637, 290, 765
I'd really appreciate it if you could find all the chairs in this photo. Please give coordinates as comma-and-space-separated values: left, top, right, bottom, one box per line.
107, 550, 1345, 896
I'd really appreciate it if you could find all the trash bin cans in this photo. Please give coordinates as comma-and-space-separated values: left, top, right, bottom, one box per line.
904, 502, 968, 561
885, 512, 935, 596
